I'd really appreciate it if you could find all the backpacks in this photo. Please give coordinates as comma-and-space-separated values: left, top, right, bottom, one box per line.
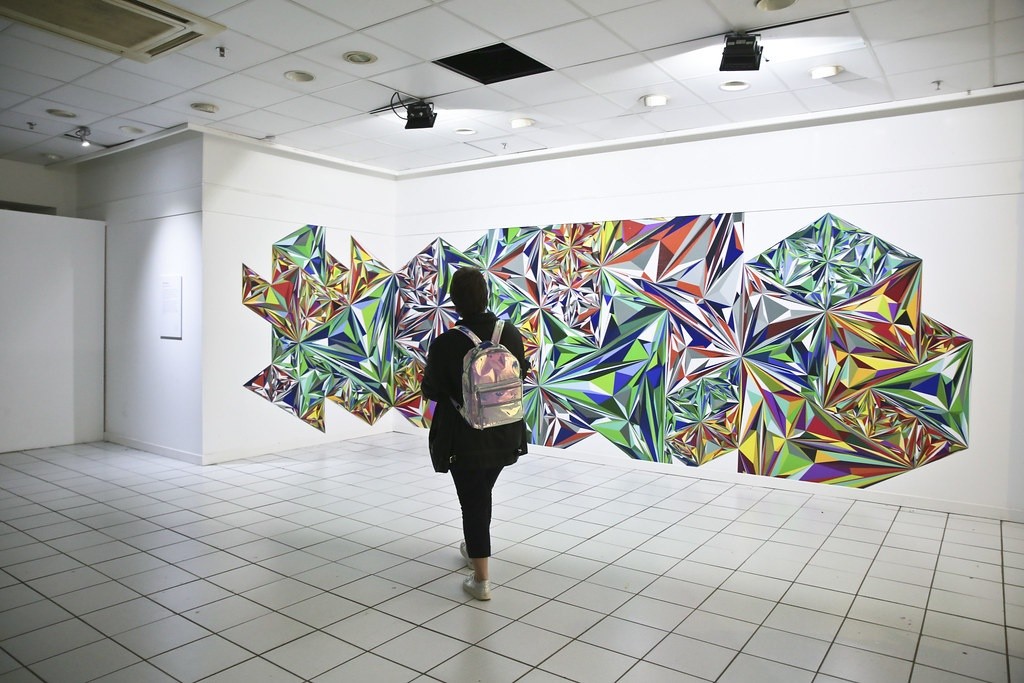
448, 318, 524, 430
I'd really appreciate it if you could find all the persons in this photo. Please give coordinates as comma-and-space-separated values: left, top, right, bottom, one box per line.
421, 267, 530, 601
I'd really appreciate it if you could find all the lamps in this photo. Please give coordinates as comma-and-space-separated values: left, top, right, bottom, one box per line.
405, 101, 437, 129
720, 33, 763, 72
80, 136, 90, 147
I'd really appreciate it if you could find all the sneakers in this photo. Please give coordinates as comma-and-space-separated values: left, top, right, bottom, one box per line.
459, 541, 474, 570
462, 570, 492, 603
